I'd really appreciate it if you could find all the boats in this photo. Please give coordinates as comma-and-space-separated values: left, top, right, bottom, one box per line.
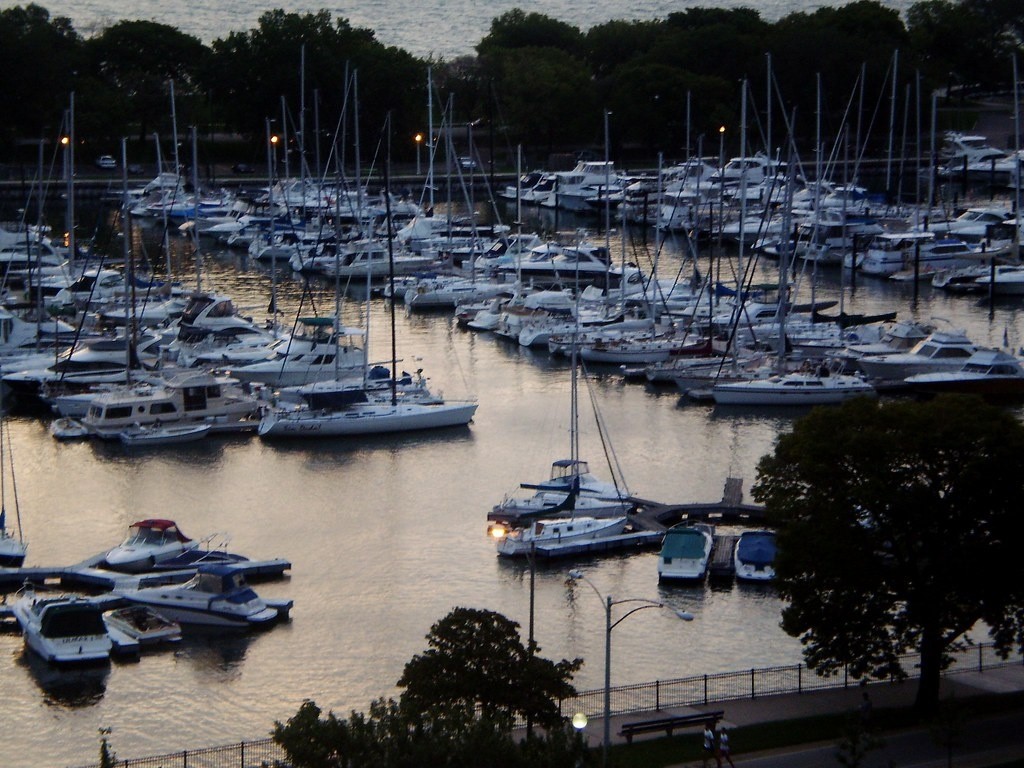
0, 397, 279, 667
654, 518, 715, 588
920, 128, 1024, 187
500, 150, 1024, 304
497, 516, 630, 556
537, 455, 629, 502
734, 532, 780, 582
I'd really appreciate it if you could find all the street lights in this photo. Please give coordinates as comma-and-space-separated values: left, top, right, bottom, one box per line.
566, 569, 696, 768
571, 712, 589, 740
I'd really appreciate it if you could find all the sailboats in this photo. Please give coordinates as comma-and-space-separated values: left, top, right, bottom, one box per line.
105, 34, 1024, 405
0, 48, 480, 442
484, 227, 635, 536
